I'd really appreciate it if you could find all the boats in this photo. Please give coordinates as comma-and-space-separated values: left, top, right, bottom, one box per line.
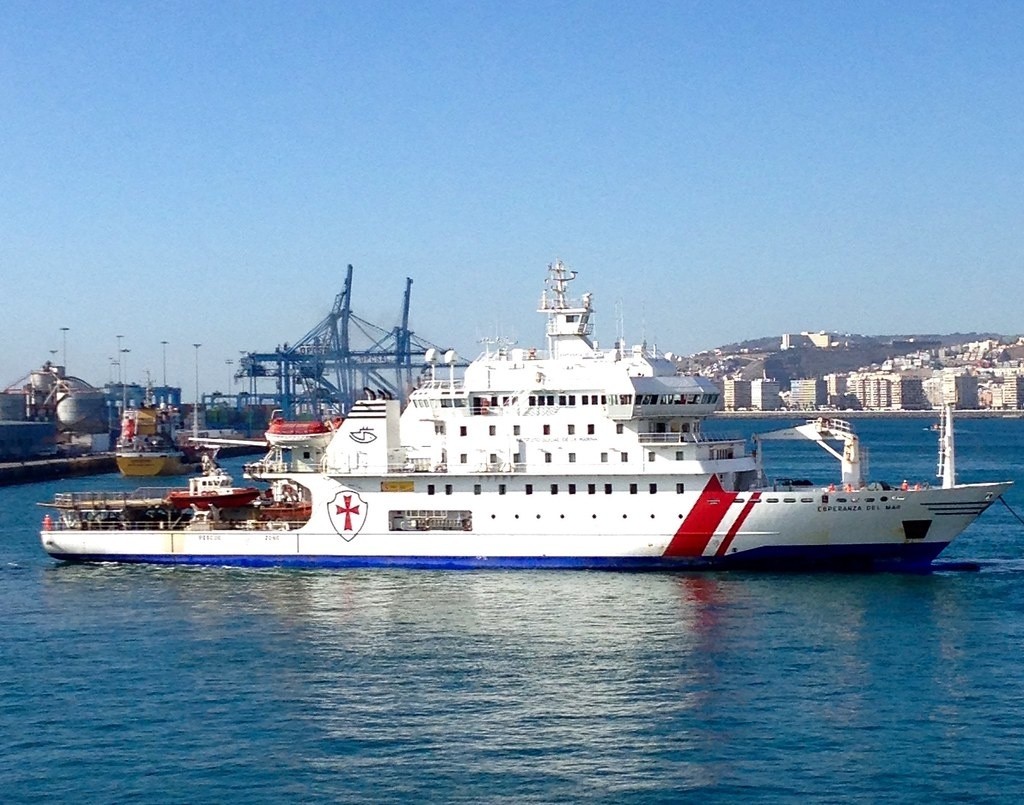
35, 254, 1014, 575
264, 418, 342, 441
115, 370, 186, 477
242, 442, 323, 480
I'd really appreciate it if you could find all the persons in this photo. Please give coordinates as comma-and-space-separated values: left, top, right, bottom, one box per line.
727, 442, 736, 459
828, 482, 855, 493
901, 478, 930, 491
44, 514, 54, 531
750, 440, 758, 458
